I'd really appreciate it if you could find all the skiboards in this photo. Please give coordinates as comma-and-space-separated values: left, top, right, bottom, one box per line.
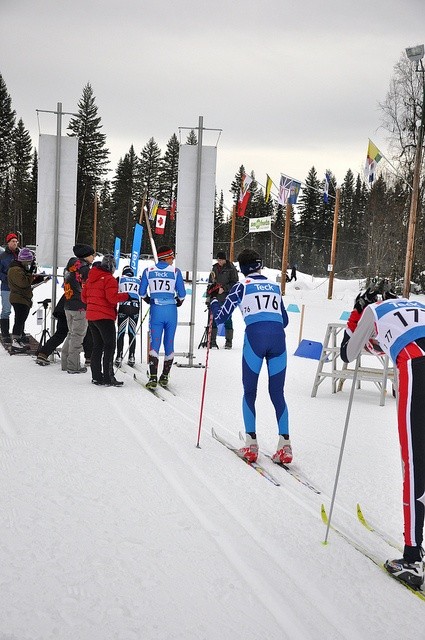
146, 370, 176, 397
320, 503, 425, 603
210, 428, 282, 487
238, 430, 321, 495
133, 373, 165, 402
124, 362, 143, 373
355, 503, 425, 560
114, 362, 127, 374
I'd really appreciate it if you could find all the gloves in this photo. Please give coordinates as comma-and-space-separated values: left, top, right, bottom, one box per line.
143, 294, 151, 304
353, 287, 379, 315
127, 294, 132, 301
381, 288, 398, 301
174, 296, 184, 307
208, 281, 219, 299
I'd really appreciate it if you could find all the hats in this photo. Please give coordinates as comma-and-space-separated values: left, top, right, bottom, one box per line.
17, 247, 33, 262
216, 252, 226, 260
364, 339, 385, 355
6, 233, 18, 244
122, 266, 134, 275
101, 255, 116, 274
157, 246, 174, 260
73, 243, 95, 259
237, 248, 263, 275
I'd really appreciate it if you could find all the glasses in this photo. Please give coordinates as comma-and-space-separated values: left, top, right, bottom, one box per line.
249, 258, 263, 271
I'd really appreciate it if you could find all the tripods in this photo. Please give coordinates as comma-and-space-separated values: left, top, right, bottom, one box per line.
36, 309, 60, 358
198, 325, 219, 349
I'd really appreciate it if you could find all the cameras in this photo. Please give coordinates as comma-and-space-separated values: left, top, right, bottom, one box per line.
37, 299, 51, 309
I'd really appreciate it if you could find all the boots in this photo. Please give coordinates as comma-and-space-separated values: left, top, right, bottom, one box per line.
210, 327, 217, 347
225, 328, 233, 349
0, 318, 12, 343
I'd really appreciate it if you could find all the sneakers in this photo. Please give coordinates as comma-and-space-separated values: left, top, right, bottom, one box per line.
67, 367, 87, 374
36, 353, 50, 364
385, 558, 425, 586
146, 375, 158, 390
104, 377, 123, 387
12, 339, 23, 348
116, 357, 122, 363
128, 357, 135, 363
273, 445, 292, 463
92, 373, 105, 385
238, 448, 258, 463
158, 374, 168, 387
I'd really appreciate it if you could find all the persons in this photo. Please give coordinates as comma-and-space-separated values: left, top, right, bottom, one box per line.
207, 250, 239, 350
289, 265, 298, 282
0, 233, 26, 341
114, 265, 142, 369
35, 292, 93, 368
61, 244, 95, 374
139, 246, 186, 391
6, 250, 49, 351
340, 287, 424, 581
81, 254, 131, 386
210, 250, 294, 468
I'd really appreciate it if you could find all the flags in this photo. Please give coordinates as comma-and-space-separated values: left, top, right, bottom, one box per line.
325, 171, 330, 204
170, 200, 175, 221
365, 140, 382, 183
154, 208, 168, 234
278, 175, 300, 205
264, 175, 273, 203
238, 171, 252, 216
146, 197, 159, 221
